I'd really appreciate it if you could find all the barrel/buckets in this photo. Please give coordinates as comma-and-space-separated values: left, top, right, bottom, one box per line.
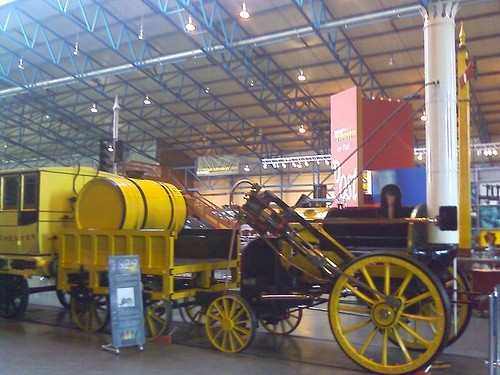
75, 176, 188, 233
